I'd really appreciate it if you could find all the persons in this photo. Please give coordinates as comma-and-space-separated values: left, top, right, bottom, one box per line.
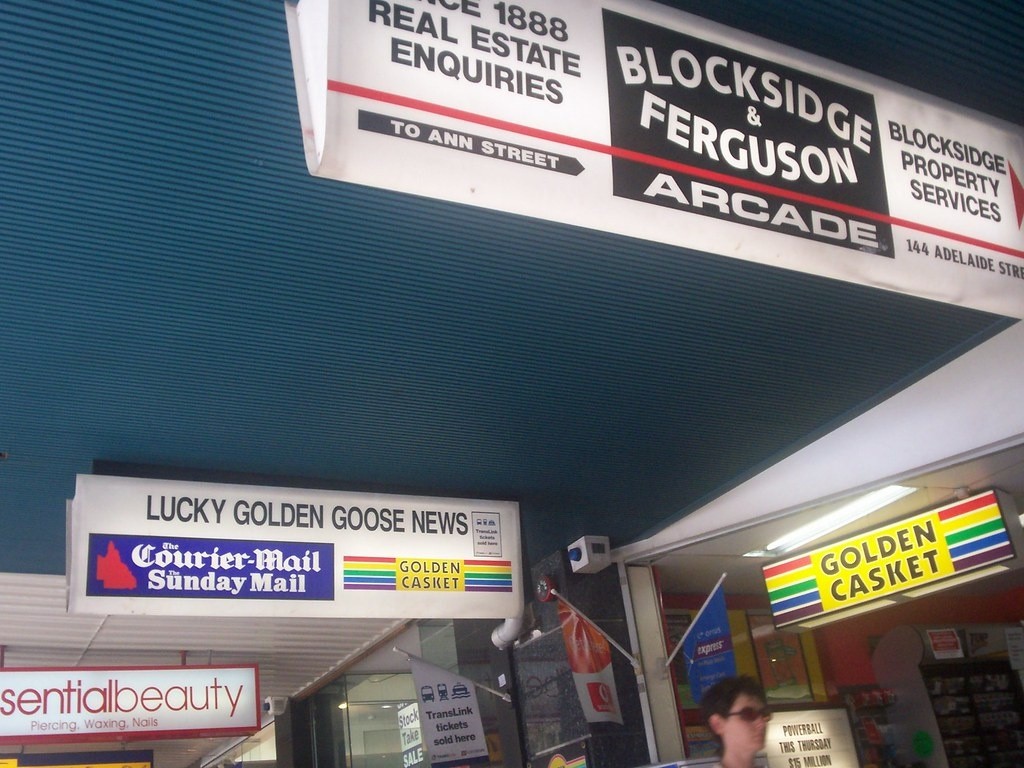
699, 676, 770, 768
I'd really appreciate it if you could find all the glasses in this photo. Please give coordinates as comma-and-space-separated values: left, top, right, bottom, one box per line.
727, 706, 773, 723
